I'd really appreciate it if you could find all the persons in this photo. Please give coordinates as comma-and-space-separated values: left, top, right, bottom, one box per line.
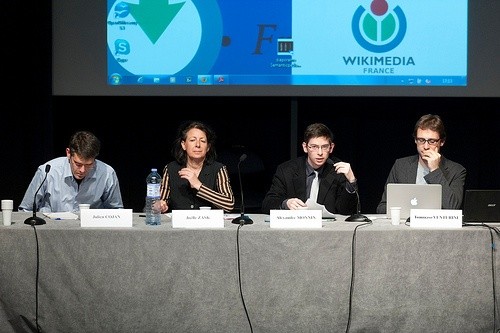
152, 121, 235, 214
376, 114, 467, 214
18, 131, 123, 213
262, 123, 359, 216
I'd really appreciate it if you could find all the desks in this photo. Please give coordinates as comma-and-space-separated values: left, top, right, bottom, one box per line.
0, 211, 500, 333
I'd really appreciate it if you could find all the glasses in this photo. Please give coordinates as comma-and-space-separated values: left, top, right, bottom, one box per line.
74, 159, 94, 168
415, 137, 440, 146
307, 144, 329, 151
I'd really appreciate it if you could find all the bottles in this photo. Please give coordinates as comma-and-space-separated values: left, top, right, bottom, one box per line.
145, 168, 162, 226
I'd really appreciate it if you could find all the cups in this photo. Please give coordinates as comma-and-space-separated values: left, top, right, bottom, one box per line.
78, 203, 90, 220
390, 207, 401, 226
1, 200, 13, 226
199, 206, 212, 210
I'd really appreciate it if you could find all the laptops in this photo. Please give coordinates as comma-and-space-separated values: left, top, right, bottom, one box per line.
386, 182, 442, 220
462, 190, 500, 223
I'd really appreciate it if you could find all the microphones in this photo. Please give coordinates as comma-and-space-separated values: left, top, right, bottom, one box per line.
237, 154, 247, 220
33, 164, 50, 219
326, 159, 360, 215
